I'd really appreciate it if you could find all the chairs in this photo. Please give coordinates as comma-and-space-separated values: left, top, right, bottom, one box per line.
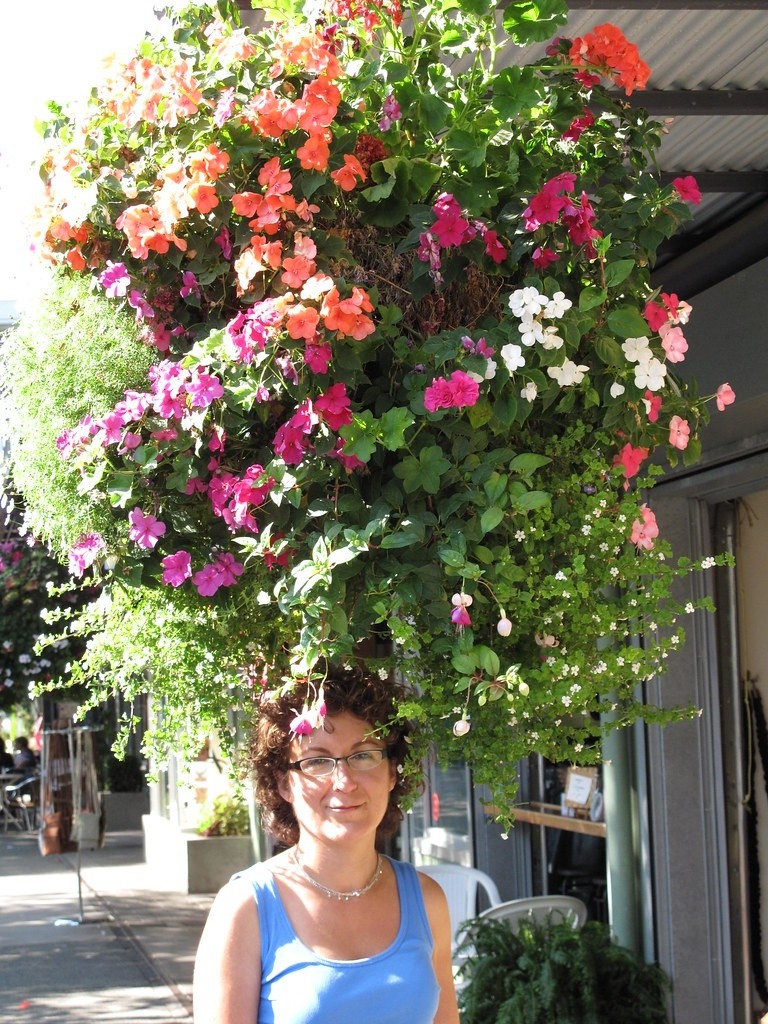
414, 865, 587, 994
4, 777, 41, 833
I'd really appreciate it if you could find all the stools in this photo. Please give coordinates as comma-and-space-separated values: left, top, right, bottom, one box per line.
556, 867, 608, 926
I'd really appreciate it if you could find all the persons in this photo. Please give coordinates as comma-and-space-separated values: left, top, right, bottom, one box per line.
0, 736, 35, 818
194, 666, 461, 1024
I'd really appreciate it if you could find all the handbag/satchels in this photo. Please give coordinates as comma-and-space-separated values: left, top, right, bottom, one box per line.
36, 731, 105, 857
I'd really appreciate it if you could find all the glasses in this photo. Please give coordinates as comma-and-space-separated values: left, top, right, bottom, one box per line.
282, 747, 389, 776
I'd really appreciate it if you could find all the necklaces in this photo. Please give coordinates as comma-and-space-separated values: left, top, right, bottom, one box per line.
289, 846, 383, 901
